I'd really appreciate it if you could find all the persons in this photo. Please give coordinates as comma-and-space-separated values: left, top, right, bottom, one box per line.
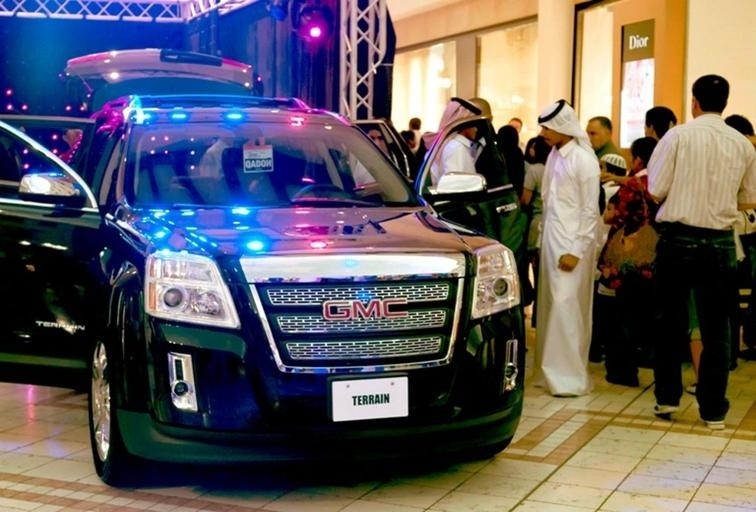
57, 128, 83, 164
644, 73, 755, 431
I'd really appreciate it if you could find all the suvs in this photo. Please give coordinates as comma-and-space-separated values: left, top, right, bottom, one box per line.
0, 45, 526, 488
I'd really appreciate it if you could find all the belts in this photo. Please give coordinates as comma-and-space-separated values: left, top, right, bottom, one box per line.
661, 221, 734, 238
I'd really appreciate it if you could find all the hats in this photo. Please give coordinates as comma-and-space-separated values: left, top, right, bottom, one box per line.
600, 153, 627, 169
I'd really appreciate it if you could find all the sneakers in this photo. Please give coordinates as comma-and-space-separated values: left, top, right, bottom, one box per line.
653, 403, 680, 414
685, 382, 697, 395
699, 410, 725, 430
